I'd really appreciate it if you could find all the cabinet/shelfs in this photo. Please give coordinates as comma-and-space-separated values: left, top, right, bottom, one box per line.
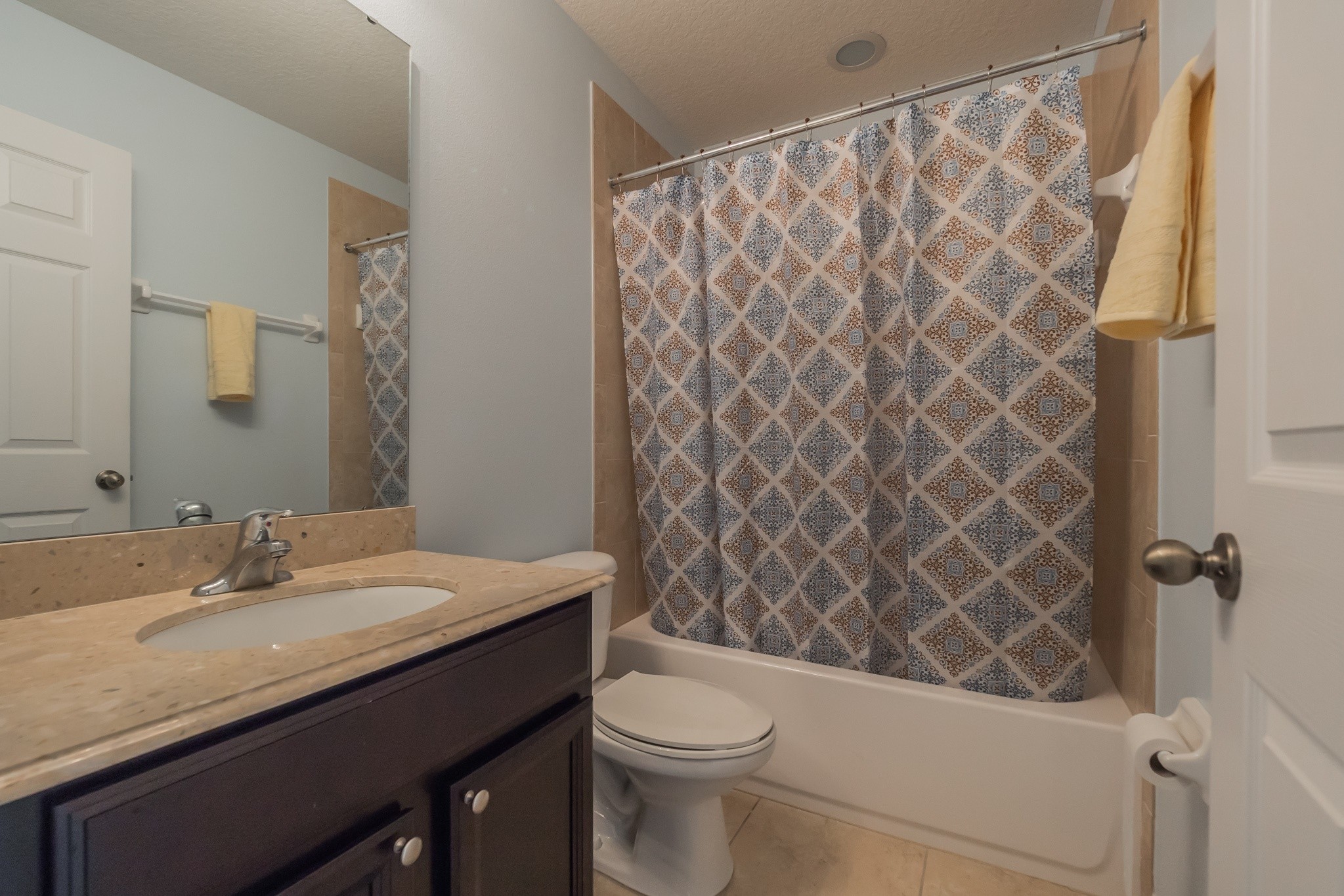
0, 592, 596, 896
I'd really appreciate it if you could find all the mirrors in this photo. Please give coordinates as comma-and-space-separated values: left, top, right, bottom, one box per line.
0, 0, 409, 544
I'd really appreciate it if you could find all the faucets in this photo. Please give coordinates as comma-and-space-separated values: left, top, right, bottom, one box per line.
192, 507, 295, 598
173, 497, 214, 525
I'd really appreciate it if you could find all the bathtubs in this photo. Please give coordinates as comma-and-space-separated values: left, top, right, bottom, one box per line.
608, 605, 1137, 894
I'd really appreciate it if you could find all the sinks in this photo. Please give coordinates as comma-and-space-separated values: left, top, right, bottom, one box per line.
133, 574, 460, 656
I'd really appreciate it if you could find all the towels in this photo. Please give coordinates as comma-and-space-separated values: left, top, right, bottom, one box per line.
1089, 56, 1221, 348
202, 300, 261, 404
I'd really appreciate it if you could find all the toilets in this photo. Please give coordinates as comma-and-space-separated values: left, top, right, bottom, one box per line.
527, 547, 781, 896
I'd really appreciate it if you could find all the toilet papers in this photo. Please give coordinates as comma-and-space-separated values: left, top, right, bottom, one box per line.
1127, 712, 1192, 788
